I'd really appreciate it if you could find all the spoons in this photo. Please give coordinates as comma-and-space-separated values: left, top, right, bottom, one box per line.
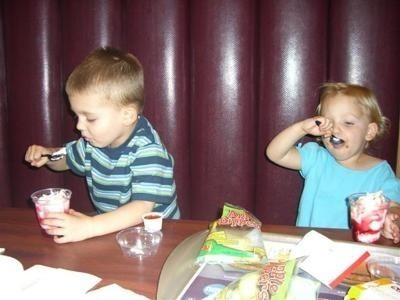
315, 120, 344, 145
38, 152, 66, 162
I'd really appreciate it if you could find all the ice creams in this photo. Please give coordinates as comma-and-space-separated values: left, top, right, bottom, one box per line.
349, 191, 387, 243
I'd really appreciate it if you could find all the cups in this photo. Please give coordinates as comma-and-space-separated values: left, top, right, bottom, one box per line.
347, 192, 391, 243
143, 212, 162, 231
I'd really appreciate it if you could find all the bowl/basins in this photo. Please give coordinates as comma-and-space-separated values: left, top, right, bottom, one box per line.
366, 255, 400, 285
115, 228, 163, 258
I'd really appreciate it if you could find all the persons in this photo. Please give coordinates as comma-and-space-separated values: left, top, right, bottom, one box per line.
25, 46, 181, 244
265, 82, 399, 244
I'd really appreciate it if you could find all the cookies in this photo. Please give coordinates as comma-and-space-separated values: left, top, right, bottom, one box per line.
342, 262, 394, 285
